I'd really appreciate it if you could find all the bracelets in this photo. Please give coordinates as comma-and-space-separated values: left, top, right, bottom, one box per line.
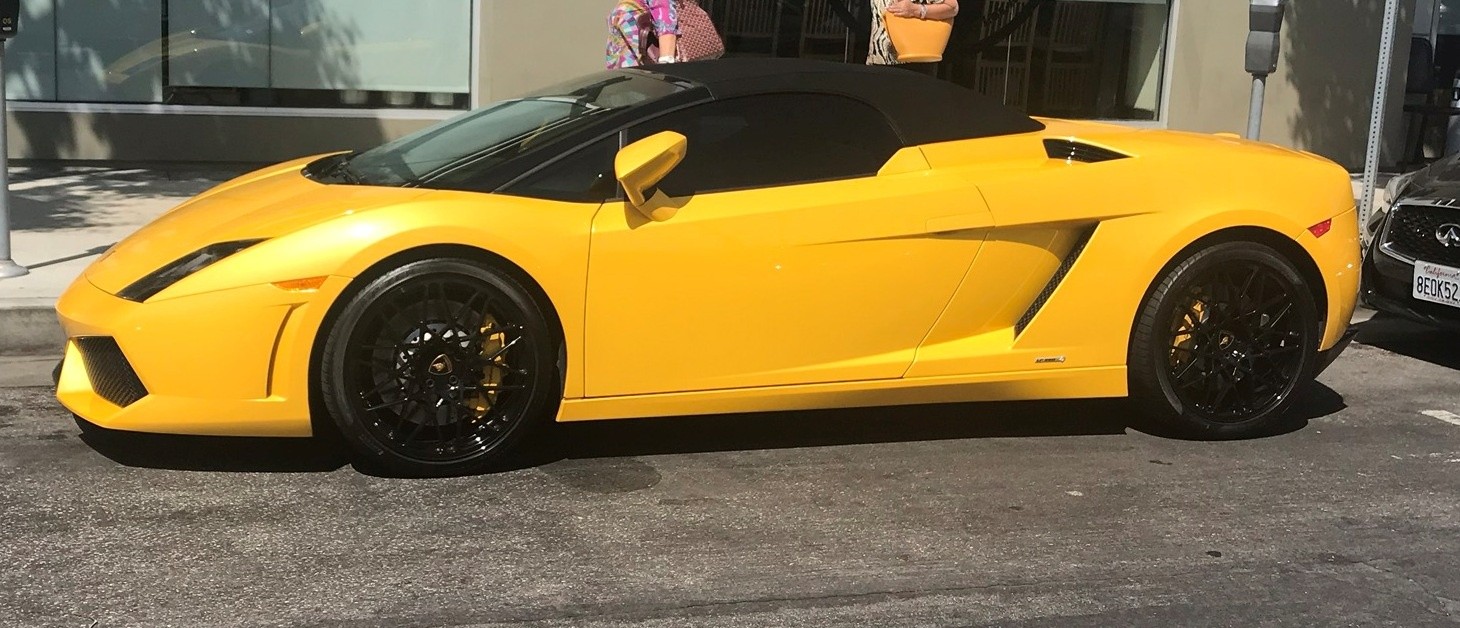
920, 3, 927, 20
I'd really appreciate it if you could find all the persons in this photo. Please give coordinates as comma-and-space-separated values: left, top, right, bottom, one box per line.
605, 0, 679, 70
864, 0, 960, 78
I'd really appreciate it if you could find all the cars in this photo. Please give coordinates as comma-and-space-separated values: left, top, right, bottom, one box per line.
1359, 148, 1460, 326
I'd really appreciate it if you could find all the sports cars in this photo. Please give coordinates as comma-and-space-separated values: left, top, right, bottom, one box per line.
52, 56, 1363, 476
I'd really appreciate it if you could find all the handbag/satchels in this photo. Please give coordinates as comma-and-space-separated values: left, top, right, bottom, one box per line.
673, 0, 725, 63
882, 11, 955, 62
